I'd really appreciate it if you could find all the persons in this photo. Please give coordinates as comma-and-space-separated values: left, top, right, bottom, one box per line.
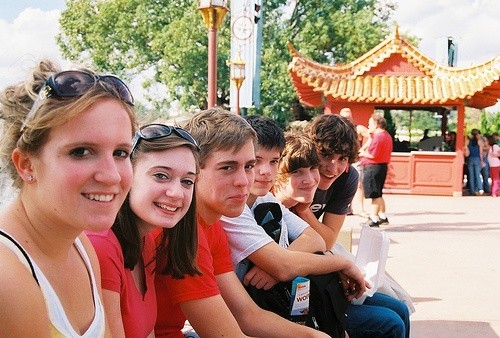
222, 117, 371, 302
338, 107, 370, 216
153, 109, 333, 338
294, 115, 360, 251
269, 130, 410, 337
416, 126, 500, 197
85, 124, 200, 338
358, 114, 393, 228
0, 59, 139, 338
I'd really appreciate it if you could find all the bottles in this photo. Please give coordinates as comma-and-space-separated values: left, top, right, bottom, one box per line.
441, 142, 445, 151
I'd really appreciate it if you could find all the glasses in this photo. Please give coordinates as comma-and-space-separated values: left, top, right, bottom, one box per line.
128, 123, 201, 158
18, 69, 136, 139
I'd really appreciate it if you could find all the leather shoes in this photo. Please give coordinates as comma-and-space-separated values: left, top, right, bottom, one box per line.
379, 217, 389, 225
360, 217, 379, 228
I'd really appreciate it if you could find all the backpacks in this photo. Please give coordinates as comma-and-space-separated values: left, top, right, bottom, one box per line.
244, 202, 348, 338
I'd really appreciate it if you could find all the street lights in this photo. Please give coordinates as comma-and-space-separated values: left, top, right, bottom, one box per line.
198, 0, 230, 111
229, 49, 246, 116
